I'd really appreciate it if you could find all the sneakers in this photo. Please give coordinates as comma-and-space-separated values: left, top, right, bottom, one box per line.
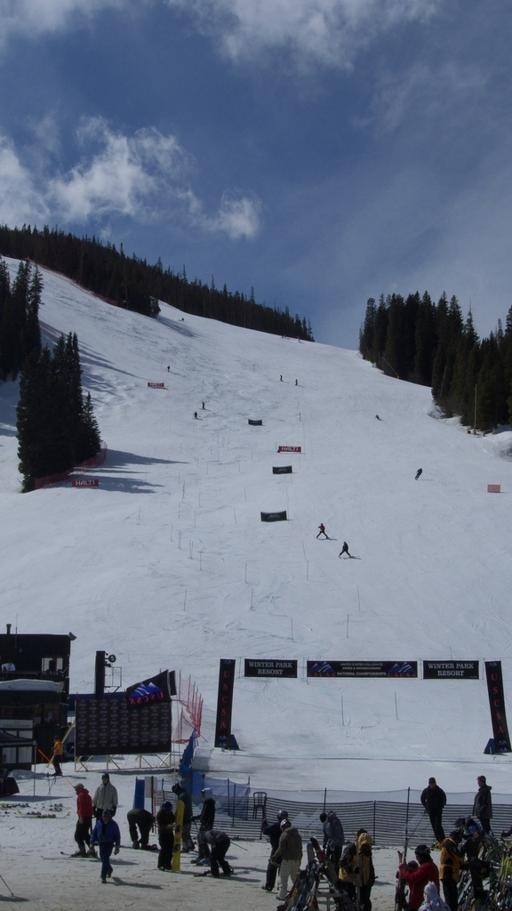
200, 858, 208, 864
107, 869, 113, 878
101, 877, 106, 883
86, 847, 96, 858
196, 856, 204, 861
207, 874, 213, 877
72, 851, 87, 858
219, 872, 230, 876
276, 896, 285, 901
180, 848, 189, 853
188, 845, 195, 849
159, 865, 172, 871
261, 885, 272, 892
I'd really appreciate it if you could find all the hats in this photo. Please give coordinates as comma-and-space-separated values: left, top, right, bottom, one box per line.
429, 777, 436, 784
450, 829, 465, 841
320, 812, 327, 822
478, 776, 486, 783
415, 844, 431, 857
356, 828, 368, 837
102, 807, 111, 817
73, 783, 84, 789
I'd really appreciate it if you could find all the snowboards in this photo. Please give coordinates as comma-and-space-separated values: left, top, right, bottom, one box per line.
171, 800, 185, 872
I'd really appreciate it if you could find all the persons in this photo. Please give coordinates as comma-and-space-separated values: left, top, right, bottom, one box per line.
197, 828, 232, 878
126, 807, 159, 851
471, 774, 494, 834
393, 819, 512, 909
194, 410, 198, 417
155, 799, 176, 872
315, 520, 332, 540
166, 364, 170, 371
73, 782, 96, 857
375, 414, 380, 419
171, 780, 195, 853
415, 467, 423, 480
294, 378, 299, 386
260, 805, 376, 910
92, 772, 117, 820
88, 809, 121, 883
201, 400, 205, 409
51, 733, 64, 775
418, 775, 449, 846
279, 373, 283, 381
190, 786, 216, 864
339, 540, 352, 558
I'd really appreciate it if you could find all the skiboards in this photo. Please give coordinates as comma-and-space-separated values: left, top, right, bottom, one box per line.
395, 850, 408, 911
41, 852, 99, 861
276, 837, 358, 911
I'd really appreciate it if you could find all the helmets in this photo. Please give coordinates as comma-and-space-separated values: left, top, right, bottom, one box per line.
277, 810, 288, 820
280, 818, 291, 828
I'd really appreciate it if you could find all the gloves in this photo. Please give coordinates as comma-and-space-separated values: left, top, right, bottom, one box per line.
88, 847, 95, 855
113, 846, 120, 855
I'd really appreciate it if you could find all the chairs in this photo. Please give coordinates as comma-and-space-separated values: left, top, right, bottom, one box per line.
252, 792, 267, 820
